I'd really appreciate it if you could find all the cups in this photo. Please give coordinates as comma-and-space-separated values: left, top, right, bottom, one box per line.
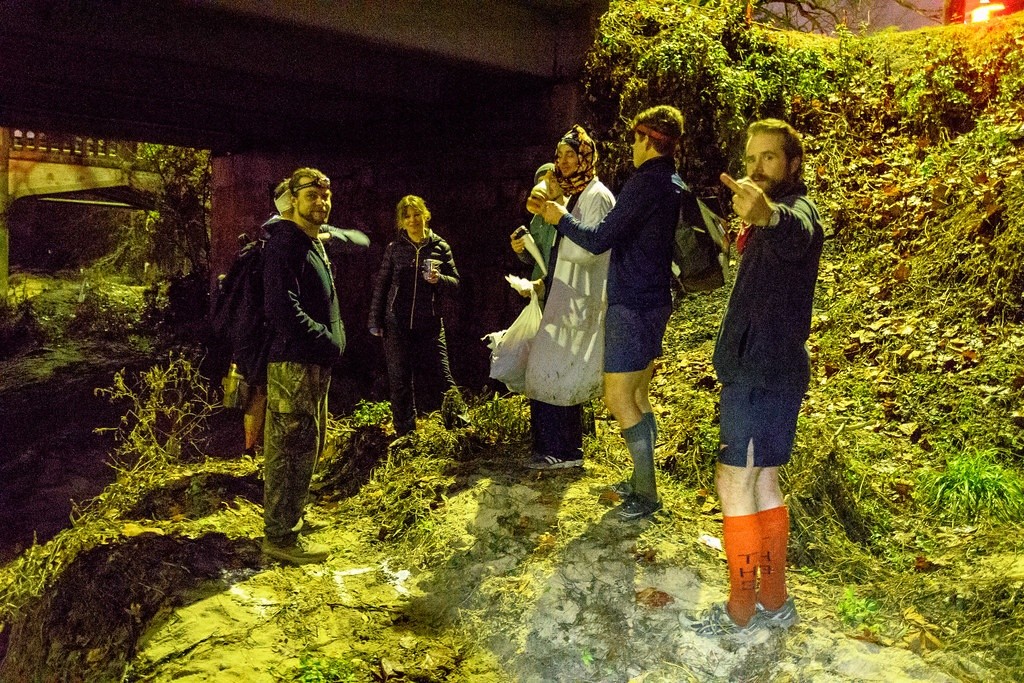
423, 260, 441, 279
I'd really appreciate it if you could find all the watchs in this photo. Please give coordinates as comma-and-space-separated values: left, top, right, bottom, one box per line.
768, 203, 780, 227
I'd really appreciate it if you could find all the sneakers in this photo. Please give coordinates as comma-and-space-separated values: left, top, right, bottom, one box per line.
529, 447, 585, 470
751, 594, 799, 628
293, 511, 329, 535
678, 601, 771, 643
261, 534, 331, 562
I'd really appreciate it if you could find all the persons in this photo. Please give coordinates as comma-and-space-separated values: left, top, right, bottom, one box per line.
510, 125, 618, 475
259, 168, 346, 563
366, 194, 461, 440
542, 105, 681, 521
678, 117, 825, 642
228, 175, 371, 500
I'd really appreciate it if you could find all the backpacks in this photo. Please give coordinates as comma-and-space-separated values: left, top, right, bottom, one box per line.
205, 231, 303, 365
673, 185, 730, 293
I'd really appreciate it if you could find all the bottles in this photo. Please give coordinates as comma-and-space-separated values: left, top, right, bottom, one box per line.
527, 170, 553, 215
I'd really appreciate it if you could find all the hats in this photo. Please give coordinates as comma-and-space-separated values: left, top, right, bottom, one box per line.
534, 162, 556, 186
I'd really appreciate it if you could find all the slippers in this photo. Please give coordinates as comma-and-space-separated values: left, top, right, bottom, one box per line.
610, 480, 636, 497
613, 490, 662, 522
